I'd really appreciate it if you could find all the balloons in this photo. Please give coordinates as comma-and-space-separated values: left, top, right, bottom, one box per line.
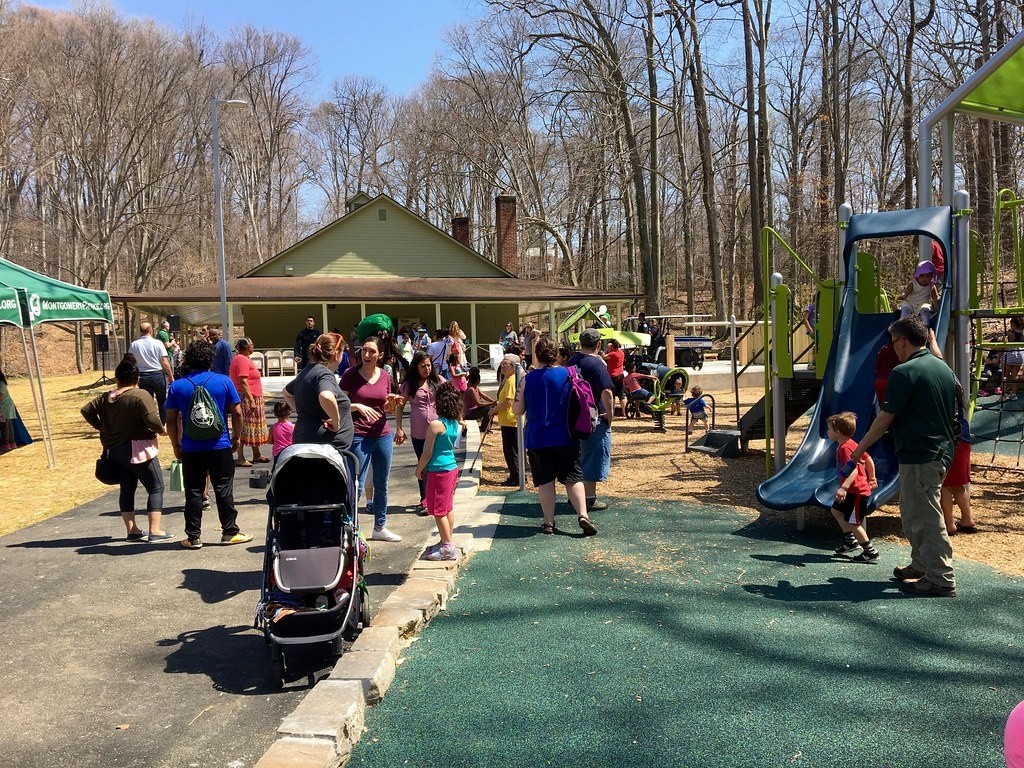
595, 304, 611, 325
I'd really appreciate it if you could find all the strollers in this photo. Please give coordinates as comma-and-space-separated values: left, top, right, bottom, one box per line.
255, 443, 371, 672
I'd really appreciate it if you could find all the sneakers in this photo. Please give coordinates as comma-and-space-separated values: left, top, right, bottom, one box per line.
125, 522, 254, 549
977, 385, 1018, 403
835, 540, 862, 554
850, 550, 880, 564
893, 564, 925, 579
364, 491, 459, 561
901, 575, 956, 597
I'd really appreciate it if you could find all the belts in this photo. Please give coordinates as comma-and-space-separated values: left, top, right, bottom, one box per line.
140, 370, 163, 375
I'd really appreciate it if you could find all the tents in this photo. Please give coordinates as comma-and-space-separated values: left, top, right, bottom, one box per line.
0, 256, 120, 470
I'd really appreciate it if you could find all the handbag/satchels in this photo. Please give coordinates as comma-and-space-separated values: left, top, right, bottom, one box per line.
95, 448, 119, 485
168, 458, 184, 491
566, 365, 599, 439
432, 361, 442, 374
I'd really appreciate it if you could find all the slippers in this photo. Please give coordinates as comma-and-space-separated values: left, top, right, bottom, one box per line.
947, 521, 977, 536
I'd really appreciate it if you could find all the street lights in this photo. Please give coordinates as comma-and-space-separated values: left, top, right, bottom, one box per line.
210, 95, 250, 343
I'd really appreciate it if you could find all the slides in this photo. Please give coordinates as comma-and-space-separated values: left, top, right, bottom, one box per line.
755, 287, 951, 514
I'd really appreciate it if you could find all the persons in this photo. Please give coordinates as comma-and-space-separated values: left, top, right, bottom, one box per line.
940, 418, 977, 535
684, 385, 712, 433
980, 337, 1004, 396
80, 320, 270, 547
284, 316, 498, 541
804, 291, 818, 369
912, 235, 944, 275
850, 314, 964, 596
512, 328, 615, 534
897, 260, 941, 327
593, 312, 660, 418
875, 323, 942, 411
671, 379, 684, 416
268, 401, 295, 457
826, 413, 881, 563
415, 383, 462, 561
997, 317, 1024, 402
488, 322, 541, 486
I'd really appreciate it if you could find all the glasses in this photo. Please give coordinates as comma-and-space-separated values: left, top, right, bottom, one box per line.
419, 331, 425, 333
506, 324, 512, 328
526, 326, 531, 328
890, 337, 908, 348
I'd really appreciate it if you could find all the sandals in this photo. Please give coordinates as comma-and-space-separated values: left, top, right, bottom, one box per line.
540, 521, 556, 534
578, 515, 598, 535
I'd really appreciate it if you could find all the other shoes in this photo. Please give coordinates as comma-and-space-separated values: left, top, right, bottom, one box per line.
234, 452, 271, 467
479, 425, 493, 434
502, 474, 528, 485
610, 403, 707, 432
567, 494, 608, 512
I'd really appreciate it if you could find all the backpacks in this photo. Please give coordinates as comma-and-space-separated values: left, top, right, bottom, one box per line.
185, 375, 224, 442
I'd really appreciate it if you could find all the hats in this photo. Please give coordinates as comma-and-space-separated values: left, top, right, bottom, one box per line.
579, 328, 601, 344
418, 324, 427, 331
914, 259, 936, 277
